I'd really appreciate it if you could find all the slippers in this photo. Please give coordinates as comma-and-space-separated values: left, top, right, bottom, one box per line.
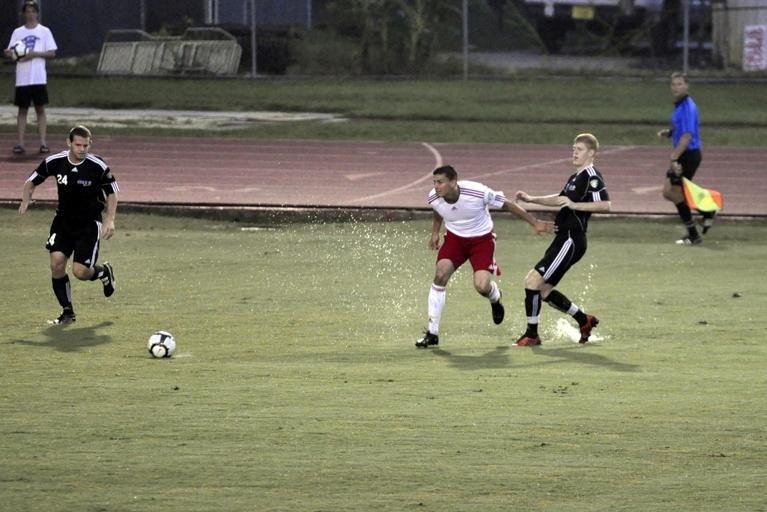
13, 145, 24, 153
40, 145, 49, 153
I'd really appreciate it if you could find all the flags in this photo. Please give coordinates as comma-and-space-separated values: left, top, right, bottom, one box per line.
682, 176, 724, 213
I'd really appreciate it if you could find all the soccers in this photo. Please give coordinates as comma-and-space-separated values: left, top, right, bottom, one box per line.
148, 331, 176, 358
10, 41, 29, 62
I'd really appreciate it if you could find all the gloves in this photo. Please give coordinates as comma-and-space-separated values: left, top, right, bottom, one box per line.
672, 159, 683, 177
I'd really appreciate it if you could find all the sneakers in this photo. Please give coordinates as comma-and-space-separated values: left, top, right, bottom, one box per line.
700, 210, 718, 234
47, 314, 76, 325
512, 334, 541, 347
416, 331, 439, 348
490, 288, 504, 324
578, 315, 599, 344
101, 261, 117, 297
674, 237, 703, 245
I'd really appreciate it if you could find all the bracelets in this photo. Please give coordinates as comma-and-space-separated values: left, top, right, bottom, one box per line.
670, 158, 678, 163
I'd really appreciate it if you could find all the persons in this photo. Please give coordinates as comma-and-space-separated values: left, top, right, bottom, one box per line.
414, 164, 559, 348
3, 0, 57, 156
513, 132, 611, 348
656, 73, 723, 247
17, 125, 118, 327
